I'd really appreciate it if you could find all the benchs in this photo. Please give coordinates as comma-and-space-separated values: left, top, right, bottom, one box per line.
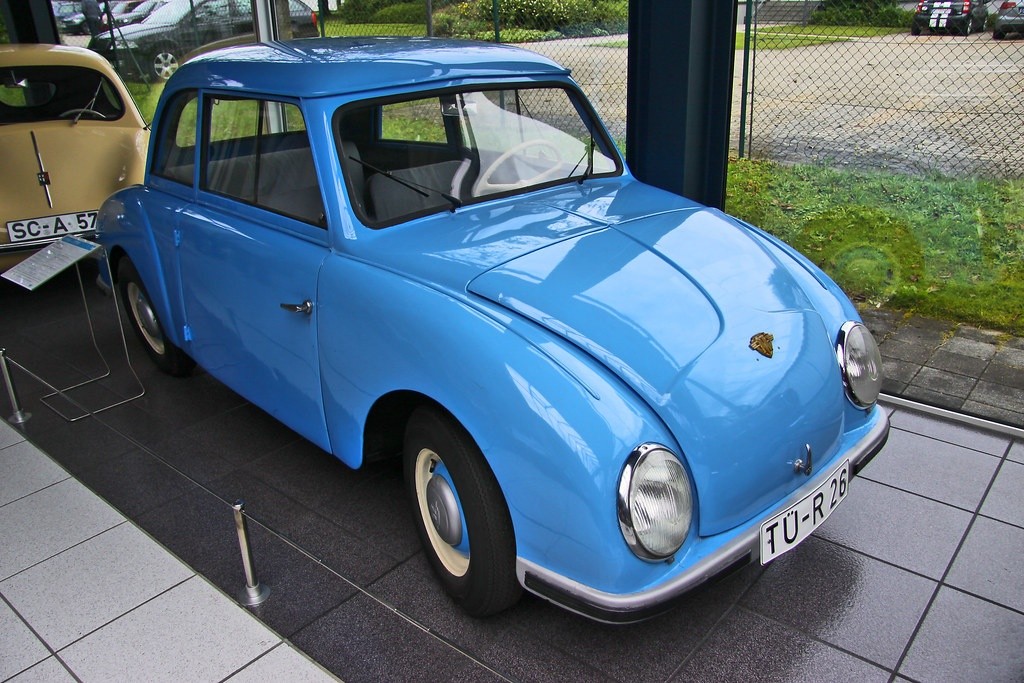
171, 140, 369, 221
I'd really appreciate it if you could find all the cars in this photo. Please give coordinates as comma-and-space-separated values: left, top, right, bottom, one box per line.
96, 36, 890, 621
993, 0, 1024, 39
0, 44, 151, 277
51, 0, 170, 36
86, 0, 321, 84
911, 0, 989, 36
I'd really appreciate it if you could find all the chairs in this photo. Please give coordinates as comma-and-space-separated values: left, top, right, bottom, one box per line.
369, 159, 470, 222
